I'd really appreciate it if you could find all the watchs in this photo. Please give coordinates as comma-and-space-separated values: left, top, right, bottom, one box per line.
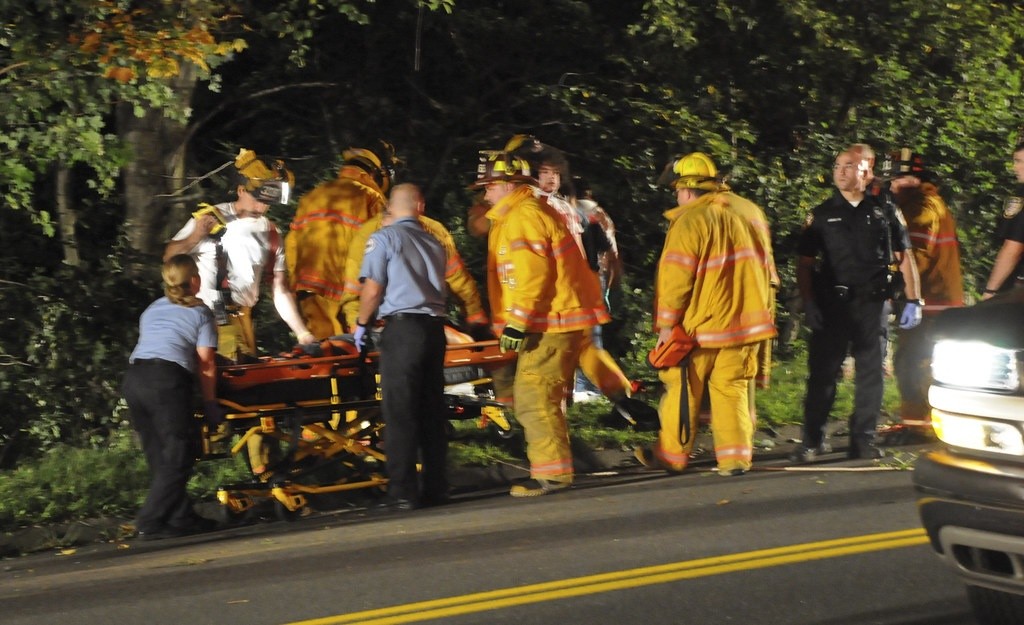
906, 298, 926, 306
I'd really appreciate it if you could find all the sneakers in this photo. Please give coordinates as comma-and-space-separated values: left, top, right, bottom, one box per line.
510, 480, 567, 496
633, 448, 681, 475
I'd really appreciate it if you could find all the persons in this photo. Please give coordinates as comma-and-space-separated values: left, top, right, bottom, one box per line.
633, 152, 780, 476
163, 132, 624, 470
466, 150, 612, 498
353, 181, 449, 510
979, 143, 1024, 303
795, 142, 964, 462
123, 254, 220, 541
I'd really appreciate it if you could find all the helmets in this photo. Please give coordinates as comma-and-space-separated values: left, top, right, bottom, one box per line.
342, 147, 389, 192
466, 150, 540, 190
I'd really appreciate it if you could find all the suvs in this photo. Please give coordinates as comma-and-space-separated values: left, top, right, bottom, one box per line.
896, 285, 1023, 625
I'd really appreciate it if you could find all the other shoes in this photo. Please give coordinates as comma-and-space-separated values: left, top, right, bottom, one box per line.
789, 439, 831, 461
376, 491, 447, 511
138, 514, 218, 540
847, 446, 883, 460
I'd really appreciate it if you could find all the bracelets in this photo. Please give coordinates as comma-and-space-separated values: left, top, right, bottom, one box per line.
981, 287, 998, 295
357, 317, 368, 327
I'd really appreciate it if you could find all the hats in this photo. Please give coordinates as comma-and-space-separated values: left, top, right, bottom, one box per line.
878, 147, 924, 182
655, 152, 729, 192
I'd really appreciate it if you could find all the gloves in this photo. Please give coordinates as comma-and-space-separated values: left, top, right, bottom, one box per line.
899, 303, 922, 329
499, 323, 525, 354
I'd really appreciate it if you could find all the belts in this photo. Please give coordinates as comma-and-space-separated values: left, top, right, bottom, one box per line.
383, 313, 436, 321
133, 358, 174, 366
